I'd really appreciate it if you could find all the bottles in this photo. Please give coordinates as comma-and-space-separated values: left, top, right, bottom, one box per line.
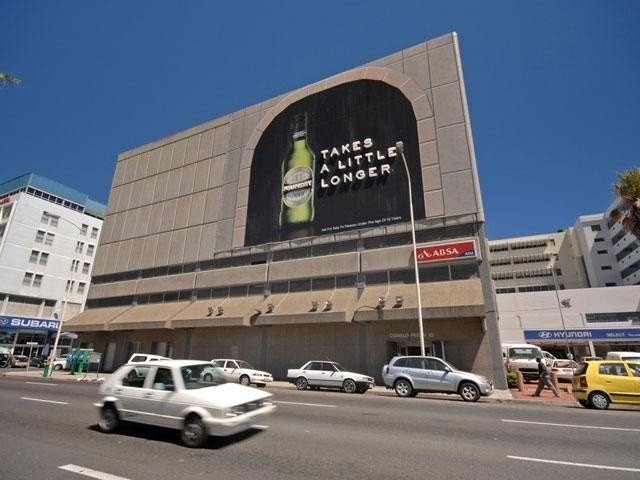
276, 111, 316, 242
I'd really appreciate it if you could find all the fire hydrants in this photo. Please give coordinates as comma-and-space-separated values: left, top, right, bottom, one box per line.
43, 364, 49, 377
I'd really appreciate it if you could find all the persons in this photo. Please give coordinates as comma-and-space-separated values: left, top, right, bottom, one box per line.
531, 356, 561, 398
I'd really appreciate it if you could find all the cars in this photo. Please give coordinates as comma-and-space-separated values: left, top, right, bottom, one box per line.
501, 342, 640, 409
92, 360, 277, 448
286, 356, 494, 401
53, 354, 68, 370
127, 353, 273, 387
0, 354, 47, 368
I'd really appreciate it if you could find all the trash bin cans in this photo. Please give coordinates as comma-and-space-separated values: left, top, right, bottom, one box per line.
44, 365, 49, 377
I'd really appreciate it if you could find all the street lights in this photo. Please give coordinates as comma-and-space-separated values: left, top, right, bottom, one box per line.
396, 141, 425, 369
43, 210, 82, 375
